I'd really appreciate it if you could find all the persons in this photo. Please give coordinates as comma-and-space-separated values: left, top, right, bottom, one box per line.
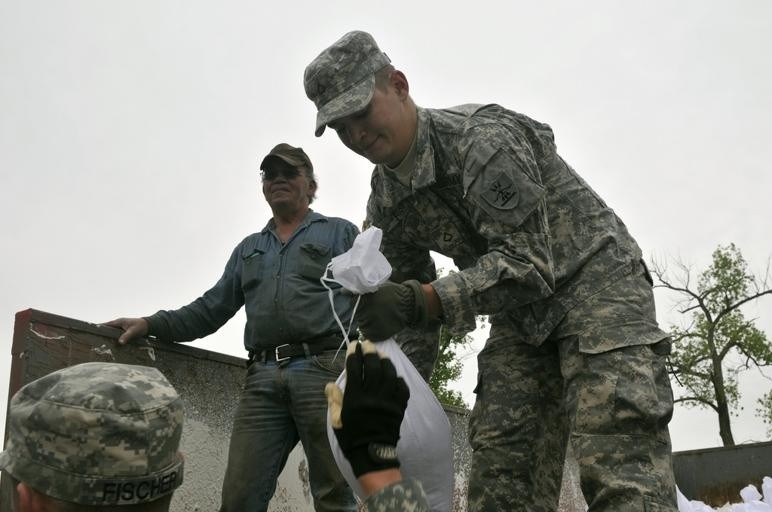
320, 338, 429, 512
102, 142, 364, 512
300, 27, 682, 512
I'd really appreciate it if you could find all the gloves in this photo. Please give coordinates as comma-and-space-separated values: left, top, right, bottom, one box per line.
325, 339, 410, 479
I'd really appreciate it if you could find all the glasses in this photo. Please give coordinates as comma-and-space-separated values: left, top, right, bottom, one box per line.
260, 169, 306, 182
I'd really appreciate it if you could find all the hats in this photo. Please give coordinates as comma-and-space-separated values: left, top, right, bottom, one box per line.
304, 30, 391, 137
260, 143, 313, 172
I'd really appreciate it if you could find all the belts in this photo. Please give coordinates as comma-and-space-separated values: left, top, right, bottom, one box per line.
258, 336, 347, 362
